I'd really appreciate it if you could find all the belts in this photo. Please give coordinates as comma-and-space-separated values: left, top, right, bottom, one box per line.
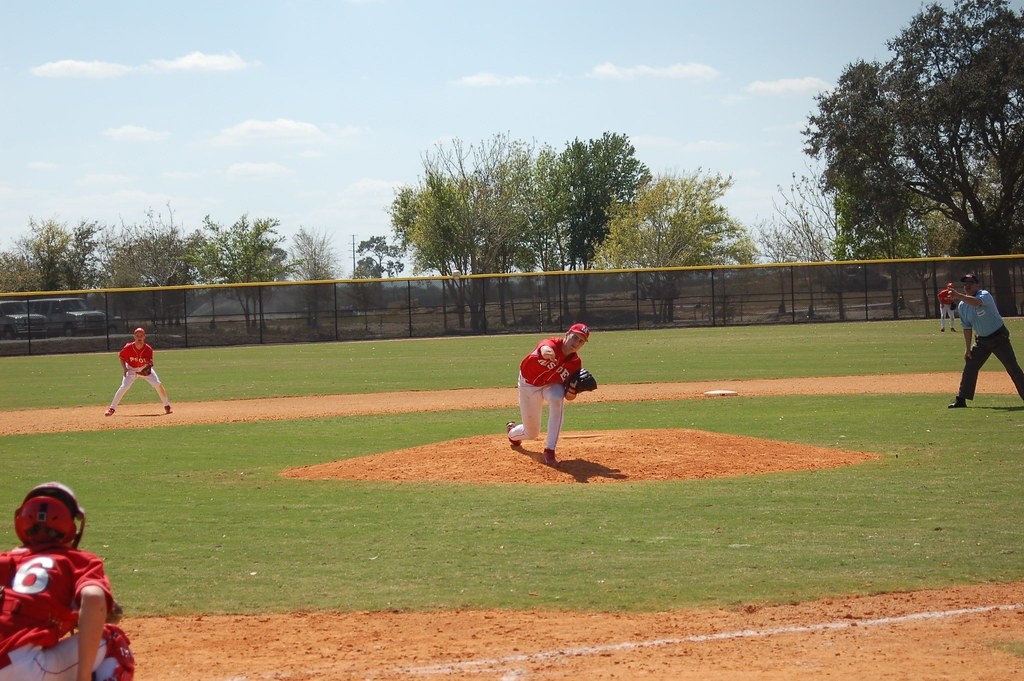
990, 326, 1004, 337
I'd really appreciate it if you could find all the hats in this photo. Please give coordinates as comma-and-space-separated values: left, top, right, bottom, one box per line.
947, 282, 954, 287
568, 323, 590, 340
960, 272, 979, 283
133, 327, 145, 336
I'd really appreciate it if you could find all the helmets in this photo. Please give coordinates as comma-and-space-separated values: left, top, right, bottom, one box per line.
13, 481, 85, 546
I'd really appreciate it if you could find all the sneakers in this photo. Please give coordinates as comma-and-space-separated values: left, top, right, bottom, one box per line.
505, 420, 522, 446
543, 448, 559, 468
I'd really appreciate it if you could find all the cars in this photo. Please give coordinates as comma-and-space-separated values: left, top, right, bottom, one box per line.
95, 309, 122, 334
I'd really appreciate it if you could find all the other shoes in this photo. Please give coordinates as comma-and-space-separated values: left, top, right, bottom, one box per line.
105, 408, 115, 417
941, 329, 945, 332
164, 404, 173, 414
948, 396, 967, 408
951, 328, 956, 332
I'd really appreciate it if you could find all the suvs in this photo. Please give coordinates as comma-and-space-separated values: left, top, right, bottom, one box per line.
22, 298, 107, 337
845, 267, 888, 292
0, 301, 49, 340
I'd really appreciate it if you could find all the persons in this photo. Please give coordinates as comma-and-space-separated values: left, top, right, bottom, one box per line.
105, 328, 173, 416
0, 482, 134, 681
948, 273, 1024, 408
507, 323, 597, 467
938, 282, 956, 331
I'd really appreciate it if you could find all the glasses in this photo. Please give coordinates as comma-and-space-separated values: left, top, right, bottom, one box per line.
963, 280, 973, 285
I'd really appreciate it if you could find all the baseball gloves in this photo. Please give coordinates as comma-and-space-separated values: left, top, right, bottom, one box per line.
566, 368, 597, 393
135, 364, 151, 376
